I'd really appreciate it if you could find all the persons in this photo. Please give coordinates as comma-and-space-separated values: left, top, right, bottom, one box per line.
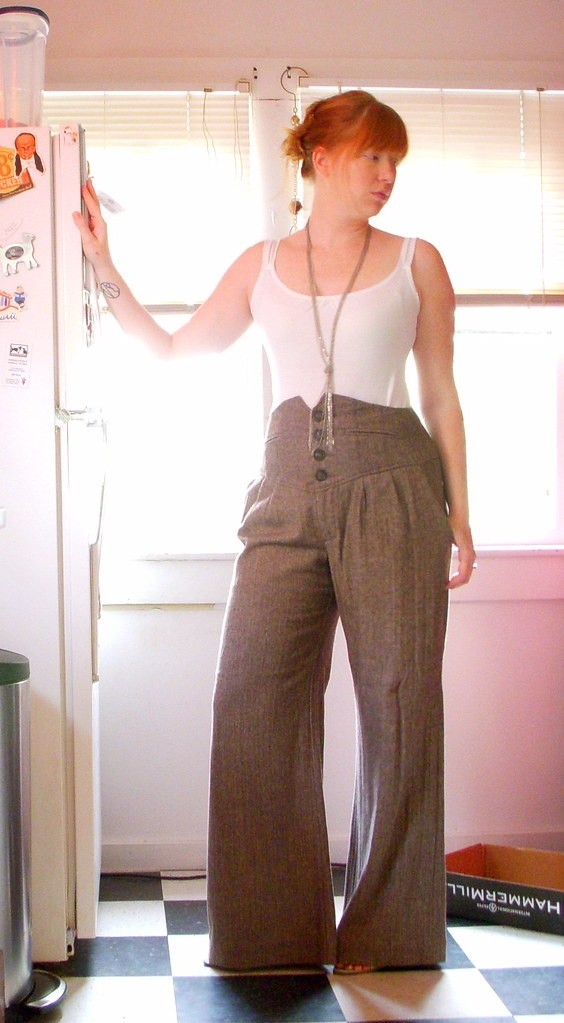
72, 90, 477, 976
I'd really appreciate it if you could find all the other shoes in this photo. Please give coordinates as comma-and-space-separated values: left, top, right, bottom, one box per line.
333, 963, 378, 974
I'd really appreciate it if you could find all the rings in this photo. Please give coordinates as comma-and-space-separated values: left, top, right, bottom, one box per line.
472, 562, 477, 569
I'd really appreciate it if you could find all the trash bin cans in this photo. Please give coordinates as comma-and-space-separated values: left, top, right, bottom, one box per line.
0, 645, 67, 1019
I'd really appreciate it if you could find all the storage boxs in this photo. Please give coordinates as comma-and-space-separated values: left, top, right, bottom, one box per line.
445, 843, 564, 937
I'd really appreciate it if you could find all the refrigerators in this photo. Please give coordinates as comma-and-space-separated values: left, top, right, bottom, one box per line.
0, 123, 100, 963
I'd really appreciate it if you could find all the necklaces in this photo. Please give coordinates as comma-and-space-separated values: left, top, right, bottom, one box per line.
290, 216, 371, 450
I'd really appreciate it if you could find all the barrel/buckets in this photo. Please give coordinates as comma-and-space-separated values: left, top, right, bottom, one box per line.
0, 7, 50, 125
